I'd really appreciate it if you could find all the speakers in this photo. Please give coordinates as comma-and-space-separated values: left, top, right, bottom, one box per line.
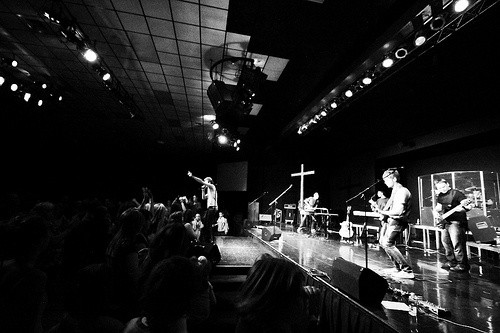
259, 214, 272, 226
331, 258, 389, 306
466, 208, 497, 243
262, 226, 282, 241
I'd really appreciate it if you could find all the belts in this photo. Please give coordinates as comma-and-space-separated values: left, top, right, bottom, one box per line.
444, 220, 466, 224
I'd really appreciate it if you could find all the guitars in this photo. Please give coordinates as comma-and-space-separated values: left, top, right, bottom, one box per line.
368, 198, 391, 224
434, 199, 471, 227
338, 206, 354, 239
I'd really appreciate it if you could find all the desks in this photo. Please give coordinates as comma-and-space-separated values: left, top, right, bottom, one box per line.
466, 241, 500, 278
409, 225, 443, 249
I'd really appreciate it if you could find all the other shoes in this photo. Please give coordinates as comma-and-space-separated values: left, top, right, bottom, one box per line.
393, 269, 415, 279
297, 227, 302, 234
450, 264, 470, 272
311, 229, 316, 234
384, 267, 399, 275
442, 260, 459, 268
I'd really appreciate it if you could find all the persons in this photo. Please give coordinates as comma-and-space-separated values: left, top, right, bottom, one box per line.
435, 179, 473, 271
371, 167, 415, 279
0, 187, 321, 333
297, 192, 319, 233
187, 170, 219, 245
376, 189, 388, 226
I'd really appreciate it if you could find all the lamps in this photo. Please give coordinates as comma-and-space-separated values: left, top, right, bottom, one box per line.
0, 57, 63, 107
94, 65, 111, 81
230, 137, 241, 152
297, 0, 472, 135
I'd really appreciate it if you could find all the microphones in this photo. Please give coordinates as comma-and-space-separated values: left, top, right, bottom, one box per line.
390, 167, 404, 170
